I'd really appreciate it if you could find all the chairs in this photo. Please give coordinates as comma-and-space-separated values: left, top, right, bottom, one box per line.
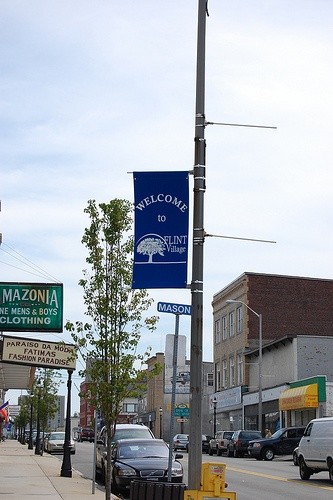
120, 447, 133, 458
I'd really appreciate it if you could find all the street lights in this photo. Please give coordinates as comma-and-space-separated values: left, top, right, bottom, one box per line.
225, 299, 263, 432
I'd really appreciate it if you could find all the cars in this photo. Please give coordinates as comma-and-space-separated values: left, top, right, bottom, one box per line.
43, 431, 77, 455
77, 428, 95, 443
185, 435, 213, 454
248, 426, 306, 461
171, 434, 190, 451
102, 438, 184, 496
169, 371, 190, 386
24, 430, 48, 450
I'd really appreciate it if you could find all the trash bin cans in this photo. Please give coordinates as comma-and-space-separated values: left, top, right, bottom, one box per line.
129, 480, 187, 500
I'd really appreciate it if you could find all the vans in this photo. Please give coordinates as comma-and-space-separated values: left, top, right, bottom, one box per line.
95, 423, 158, 483
293, 416, 333, 486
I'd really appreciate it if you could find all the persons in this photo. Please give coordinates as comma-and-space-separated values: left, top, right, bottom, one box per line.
287, 431, 293, 438
265, 429, 271, 439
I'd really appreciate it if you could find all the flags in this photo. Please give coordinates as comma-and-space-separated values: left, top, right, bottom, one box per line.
0, 401, 15, 431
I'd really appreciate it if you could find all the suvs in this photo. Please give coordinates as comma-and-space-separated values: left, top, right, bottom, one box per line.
208, 430, 236, 457
225, 429, 264, 459
